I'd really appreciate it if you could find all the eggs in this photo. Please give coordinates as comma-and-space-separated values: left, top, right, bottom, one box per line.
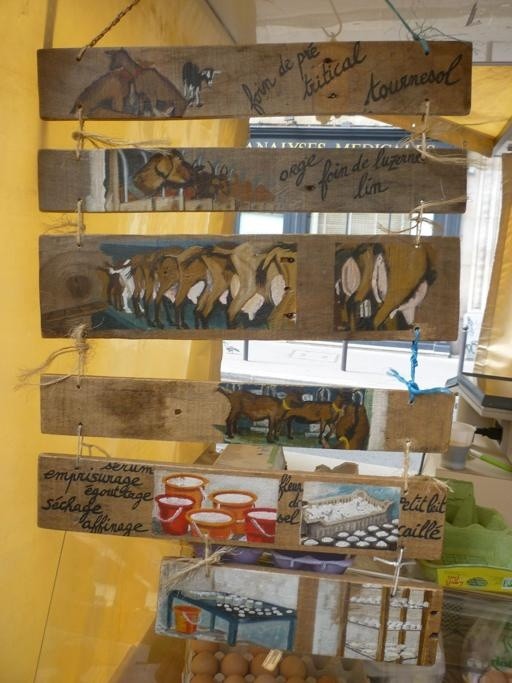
479, 670, 512, 683
189, 633, 333, 683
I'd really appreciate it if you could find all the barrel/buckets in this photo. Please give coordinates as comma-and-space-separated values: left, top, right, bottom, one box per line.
156, 493, 196, 535
186, 508, 235, 540
208, 490, 258, 535
245, 508, 278, 543
163, 474, 209, 508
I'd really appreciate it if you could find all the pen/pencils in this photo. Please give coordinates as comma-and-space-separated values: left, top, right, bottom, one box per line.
469, 449, 511, 472
